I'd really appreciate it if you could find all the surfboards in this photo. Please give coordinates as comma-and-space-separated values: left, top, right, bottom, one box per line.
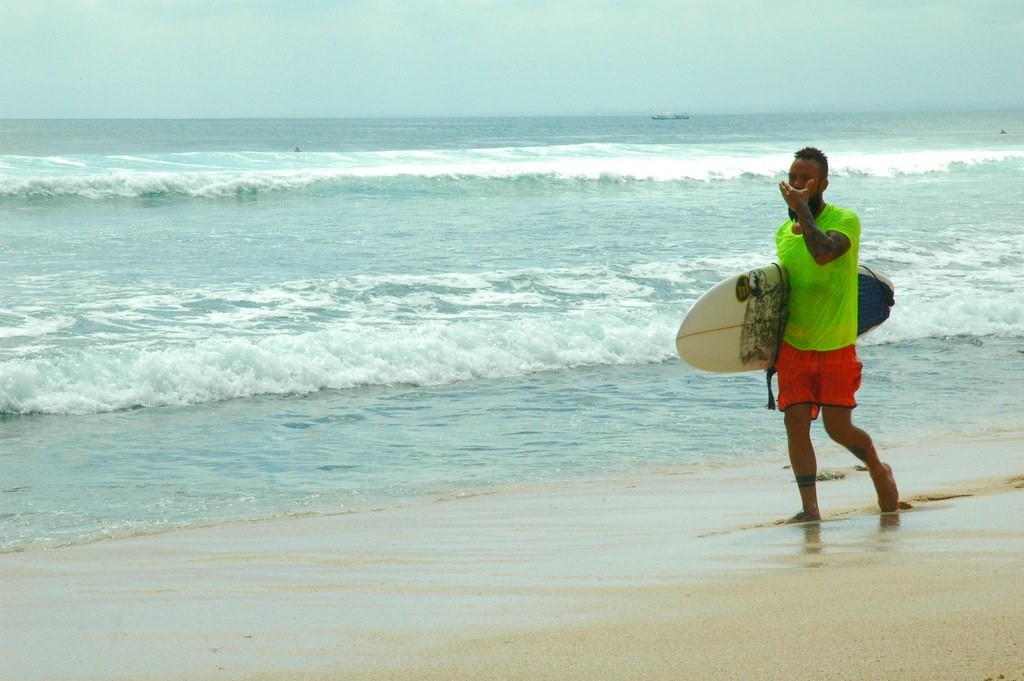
676, 262, 895, 373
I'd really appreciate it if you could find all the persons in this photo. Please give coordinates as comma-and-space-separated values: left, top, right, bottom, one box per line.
776, 147, 899, 522
